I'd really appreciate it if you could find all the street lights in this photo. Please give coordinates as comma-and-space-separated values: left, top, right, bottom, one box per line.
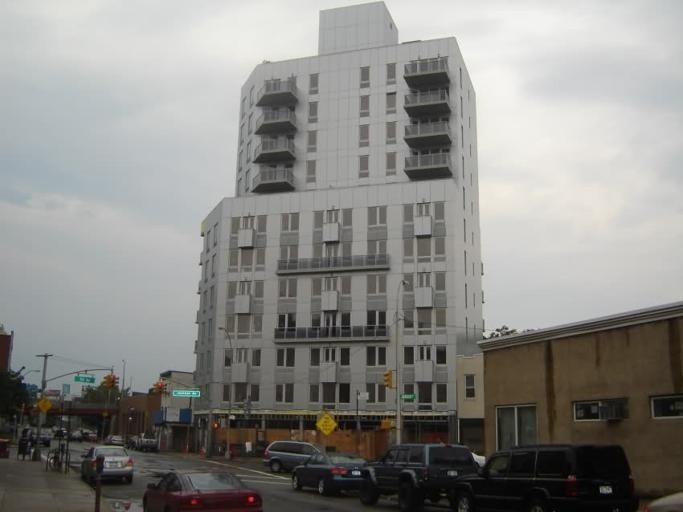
217, 326, 232, 459
22, 369, 40, 377
121, 359, 127, 398
392, 279, 410, 445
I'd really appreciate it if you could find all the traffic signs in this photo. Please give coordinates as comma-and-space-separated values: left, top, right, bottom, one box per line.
399, 393, 416, 400
74, 375, 94, 384
171, 389, 200, 398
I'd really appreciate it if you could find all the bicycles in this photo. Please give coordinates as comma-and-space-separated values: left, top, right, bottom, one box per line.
48, 442, 71, 473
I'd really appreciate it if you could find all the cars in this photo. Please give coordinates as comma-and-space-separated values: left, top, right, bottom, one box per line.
142, 468, 262, 511
470, 452, 486, 469
24, 423, 158, 454
216, 438, 269, 458
643, 492, 682, 512
290, 451, 369, 496
79, 445, 133, 486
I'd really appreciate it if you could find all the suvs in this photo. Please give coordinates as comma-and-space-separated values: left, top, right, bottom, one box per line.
262, 439, 321, 473
445, 444, 639, 511
357, 437, 480, 512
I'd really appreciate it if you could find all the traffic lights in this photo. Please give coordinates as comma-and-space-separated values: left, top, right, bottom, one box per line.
382, 369, 392, 388
152, 382, 158, 393
112, 374, 119, 390
162, 383, 167, 393
102, 374, 109, 389
108, 374, 114, 389
212, 422, 218, 430
158, 382, 162, 393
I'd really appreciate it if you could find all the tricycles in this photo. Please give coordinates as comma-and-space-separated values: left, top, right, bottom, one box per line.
16, 438, 32, 461
